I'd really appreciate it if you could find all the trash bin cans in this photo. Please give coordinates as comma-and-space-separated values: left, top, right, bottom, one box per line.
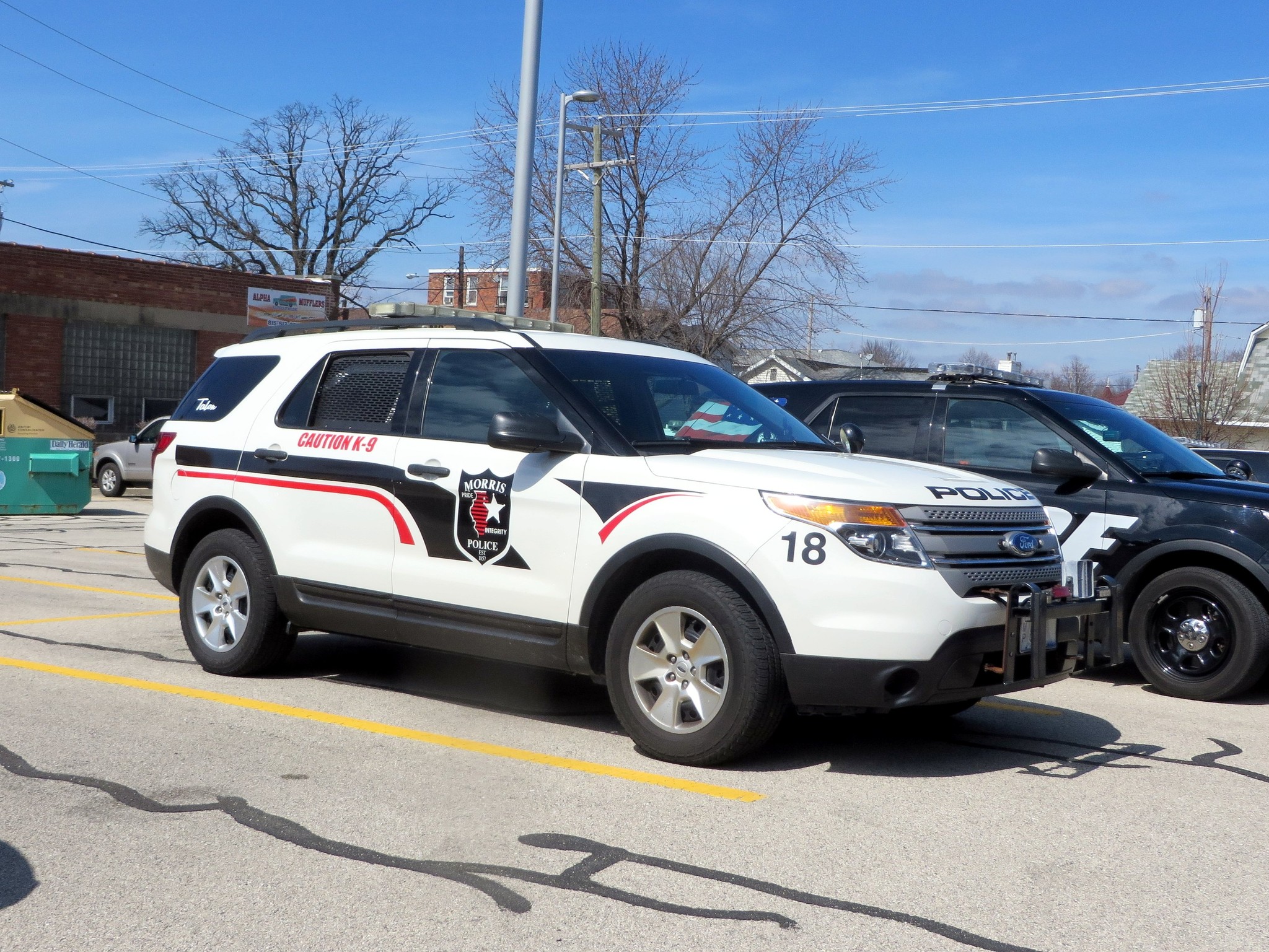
0, 388, 96, 514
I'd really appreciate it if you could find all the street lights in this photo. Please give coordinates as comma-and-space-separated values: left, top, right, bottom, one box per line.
406, 273, 464, 309
806, 326, 841, 360
549, 89, 602, 323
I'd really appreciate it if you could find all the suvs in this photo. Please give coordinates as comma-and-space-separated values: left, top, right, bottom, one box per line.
141, 314, 1127, 767
660, 361, 1269, 703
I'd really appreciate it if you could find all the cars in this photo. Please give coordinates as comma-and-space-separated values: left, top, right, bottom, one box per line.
92, 415, 171, 498
